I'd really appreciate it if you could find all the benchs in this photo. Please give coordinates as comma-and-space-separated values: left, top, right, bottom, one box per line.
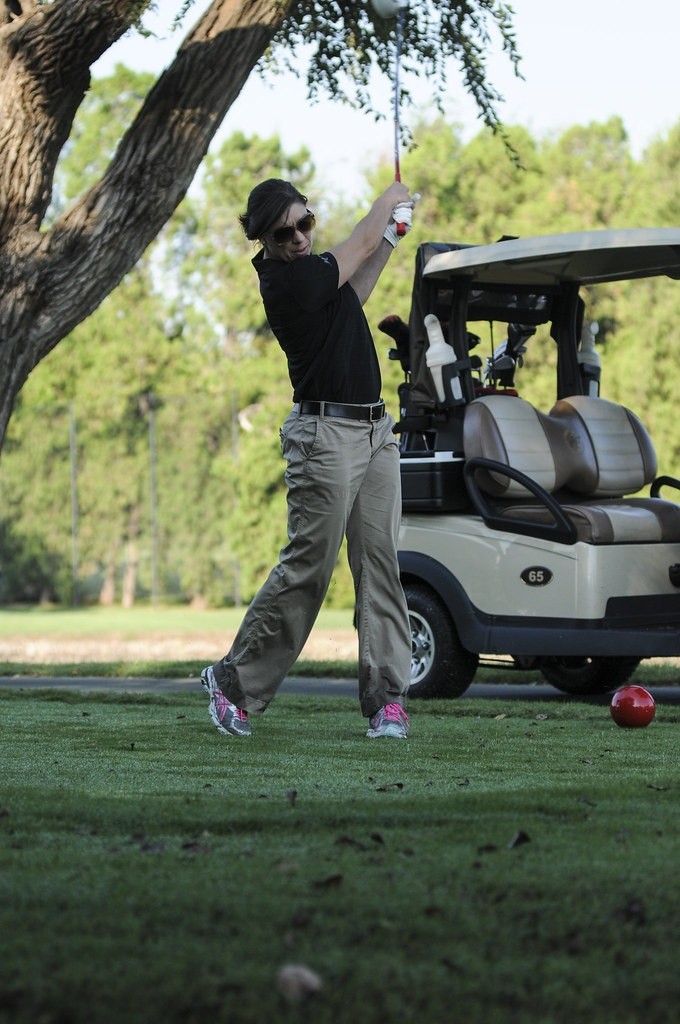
465, 395, 680, 542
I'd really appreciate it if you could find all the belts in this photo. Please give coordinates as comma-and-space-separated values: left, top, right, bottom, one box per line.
290, 400, 387, 423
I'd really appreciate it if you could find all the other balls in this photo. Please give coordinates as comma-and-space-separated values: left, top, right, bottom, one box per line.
610, 684, 657, 728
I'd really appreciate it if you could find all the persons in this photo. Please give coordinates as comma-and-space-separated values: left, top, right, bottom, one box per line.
202, 179, 421, 739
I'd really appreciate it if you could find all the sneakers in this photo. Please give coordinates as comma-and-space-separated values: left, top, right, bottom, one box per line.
201, 665, 254, 736
365, 702, 410, 738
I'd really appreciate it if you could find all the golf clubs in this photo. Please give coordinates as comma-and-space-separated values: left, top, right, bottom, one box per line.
375, 313, 537, 390
371, 0, 407, 236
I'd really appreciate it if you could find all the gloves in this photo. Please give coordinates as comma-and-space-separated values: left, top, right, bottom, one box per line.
382, 192, 422, 249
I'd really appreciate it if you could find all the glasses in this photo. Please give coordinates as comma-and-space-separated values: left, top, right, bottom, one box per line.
263, 206, 317, 247
